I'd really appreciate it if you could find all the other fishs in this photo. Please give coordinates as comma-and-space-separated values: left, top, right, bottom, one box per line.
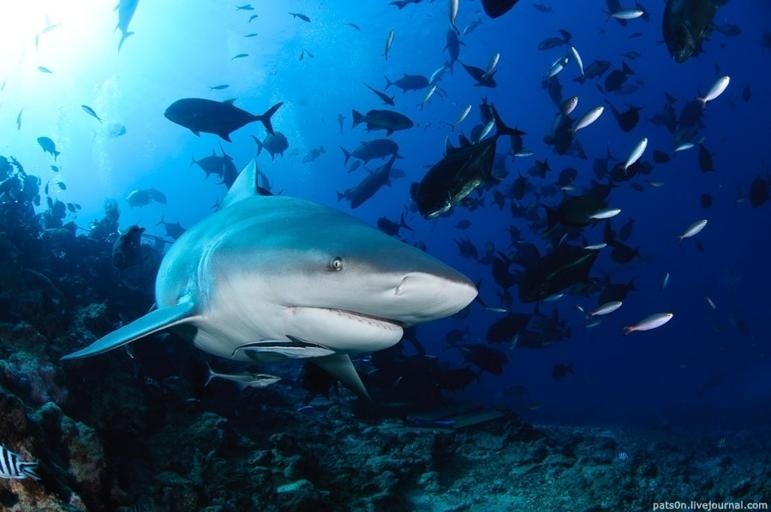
2, 0, 771, 412
